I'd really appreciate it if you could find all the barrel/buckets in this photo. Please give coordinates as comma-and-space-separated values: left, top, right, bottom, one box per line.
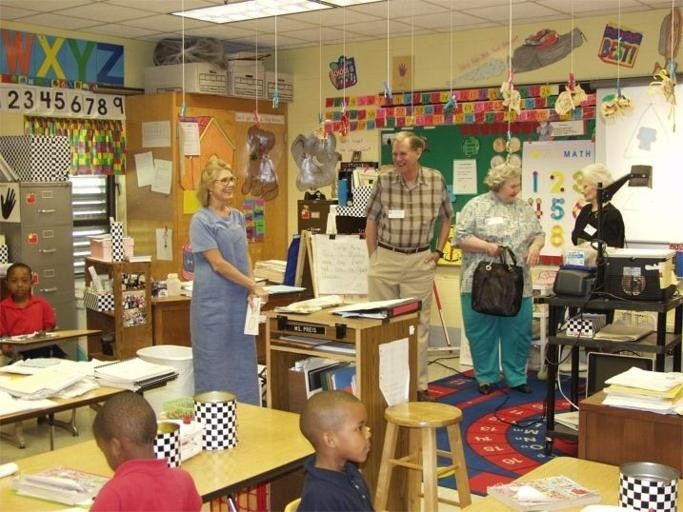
618, 462, 679, 508
137, 343, 194, 398
195, 390, 238, 450
153, 422, 182, 472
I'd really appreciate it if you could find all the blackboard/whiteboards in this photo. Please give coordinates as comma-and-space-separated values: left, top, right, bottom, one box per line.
589, 72, 683, 245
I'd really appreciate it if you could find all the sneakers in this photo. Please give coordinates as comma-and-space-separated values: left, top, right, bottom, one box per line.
417, 389, 441, 402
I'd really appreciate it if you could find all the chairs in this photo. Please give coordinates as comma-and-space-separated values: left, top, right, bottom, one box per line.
496, 285, 556, 375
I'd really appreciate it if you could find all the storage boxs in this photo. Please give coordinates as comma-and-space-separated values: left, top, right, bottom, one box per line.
263, 71, 292, 103
82, 290, 118, 313
0, 134, 73, 183
90, 235, 134, 261
140, 62, 228, 97
565, 314, 606, 340
295, 199, 338, 235
224, 66, 264, 100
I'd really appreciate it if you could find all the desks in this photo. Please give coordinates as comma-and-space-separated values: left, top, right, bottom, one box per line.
1, 400, 323, 512
0, 327, 106, 365
576, 373, 682, 478
462, 451, 682, 512
0, 358, 171, 452
150, 283, 306, 362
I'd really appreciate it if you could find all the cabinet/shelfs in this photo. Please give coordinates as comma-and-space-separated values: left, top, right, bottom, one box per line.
261, 304, 419, 510
125, 89, 290, 353
81, 256, 154, 360
0, 179, 78, 360
529, 284, 681, 457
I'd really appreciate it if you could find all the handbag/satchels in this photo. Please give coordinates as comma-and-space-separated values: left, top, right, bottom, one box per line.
471, 261, 523, 317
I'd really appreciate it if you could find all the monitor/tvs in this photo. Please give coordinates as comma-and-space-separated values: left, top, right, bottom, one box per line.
587, 352, 654, 403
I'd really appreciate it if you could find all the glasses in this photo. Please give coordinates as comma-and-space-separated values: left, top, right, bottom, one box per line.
213, 176, 240, 186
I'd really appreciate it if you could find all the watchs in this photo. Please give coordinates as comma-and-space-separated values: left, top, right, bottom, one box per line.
435, 249, 444, 258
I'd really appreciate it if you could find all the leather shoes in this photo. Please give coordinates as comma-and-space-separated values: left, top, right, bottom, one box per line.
478, 383, 492, 395
512, 384, 533, 393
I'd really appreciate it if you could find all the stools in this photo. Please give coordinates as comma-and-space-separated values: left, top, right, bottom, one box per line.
374, 400, 472, 512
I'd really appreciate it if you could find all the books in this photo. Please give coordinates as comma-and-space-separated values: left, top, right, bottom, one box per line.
10, 466, 111, 510
303, 357, 356, 402
487, 475, 601, 512
93, 357, 178, 393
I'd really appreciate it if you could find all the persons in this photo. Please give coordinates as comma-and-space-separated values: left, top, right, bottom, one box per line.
451, 163, 546, 395
0, 262, 71, 366
363, 131, 455, 403
572, 162, 625, 357
89, 391, 202, 512
189, 157, 269, 406
295, 391, 376, 512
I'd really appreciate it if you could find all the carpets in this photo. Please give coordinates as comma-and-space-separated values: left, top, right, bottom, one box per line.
406, 362, 584, 495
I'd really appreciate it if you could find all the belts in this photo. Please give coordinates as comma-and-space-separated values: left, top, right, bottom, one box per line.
377, 242, 429, 255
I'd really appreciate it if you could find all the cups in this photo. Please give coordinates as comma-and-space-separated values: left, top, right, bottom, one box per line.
337, 179, 348, 207
